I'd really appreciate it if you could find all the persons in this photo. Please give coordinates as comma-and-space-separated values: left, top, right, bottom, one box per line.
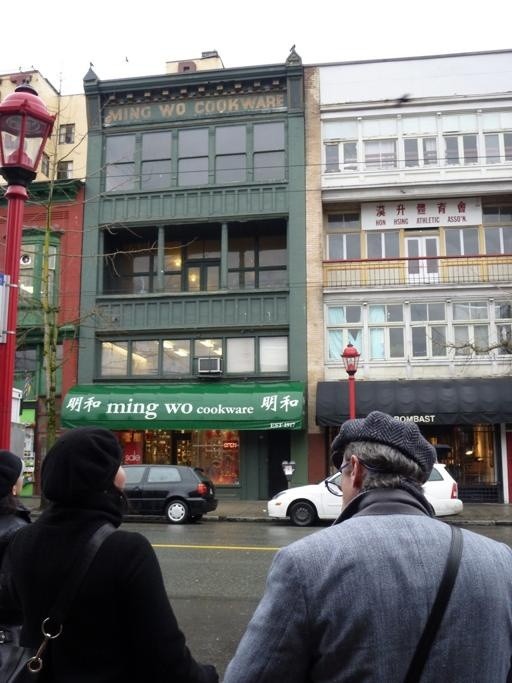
223, 410, 512, 683
0, 427, 219, 683
0, 450, 32, 551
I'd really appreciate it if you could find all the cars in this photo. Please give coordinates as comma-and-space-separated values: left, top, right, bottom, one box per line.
264, 452, 465, 526
111, 459, 220, 524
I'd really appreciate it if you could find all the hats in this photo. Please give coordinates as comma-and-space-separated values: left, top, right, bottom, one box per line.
333, 411, 439, 474
1, 452, 22, 497
39, 424, 127, 525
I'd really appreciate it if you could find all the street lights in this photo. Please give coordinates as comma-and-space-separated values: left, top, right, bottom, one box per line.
0, 76, 61, 451
340, 339, 362, 421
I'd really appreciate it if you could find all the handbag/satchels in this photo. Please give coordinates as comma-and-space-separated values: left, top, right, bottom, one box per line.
0, 624, 40, 683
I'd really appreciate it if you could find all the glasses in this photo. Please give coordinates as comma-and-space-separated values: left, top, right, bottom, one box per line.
321, 456, 372, 497
